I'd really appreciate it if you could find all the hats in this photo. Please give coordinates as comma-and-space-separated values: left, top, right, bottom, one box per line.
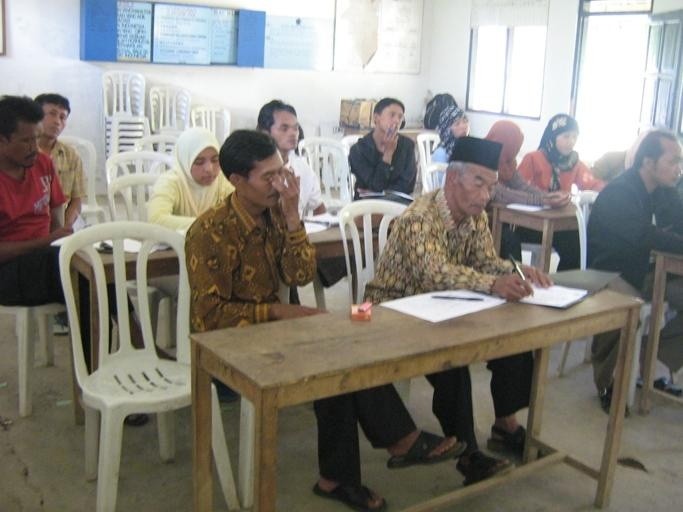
447, 136, 504, 171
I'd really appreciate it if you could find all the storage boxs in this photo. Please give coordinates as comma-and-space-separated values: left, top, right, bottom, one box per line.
339, 99, 379, 130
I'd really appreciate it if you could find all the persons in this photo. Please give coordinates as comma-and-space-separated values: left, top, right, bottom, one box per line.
0, 97, 178, 426
349, 92, 647, 273
34, 94, 88, 335
186, 129, 468, 511
587, 129, 682, 418
146, 98, 353, 405
364, 136, 556, 486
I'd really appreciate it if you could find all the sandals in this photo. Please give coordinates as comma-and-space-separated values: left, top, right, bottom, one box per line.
457, 451, 516, 486
487, 425, 546, 465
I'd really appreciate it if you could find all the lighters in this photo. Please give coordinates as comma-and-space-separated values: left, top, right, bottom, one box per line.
358, 301, 373, 312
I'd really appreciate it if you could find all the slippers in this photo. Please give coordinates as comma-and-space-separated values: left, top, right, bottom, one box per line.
313, 482, 385, 511
598, 378, 630, 418
387, 430, 467, 469
635, 377, 683, 405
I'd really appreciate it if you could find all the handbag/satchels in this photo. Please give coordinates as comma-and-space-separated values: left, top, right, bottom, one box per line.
423, 94, 456, 131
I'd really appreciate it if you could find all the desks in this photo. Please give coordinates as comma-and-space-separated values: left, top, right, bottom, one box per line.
344, 127, 434, 144
640, 248, 682, 413
69, 221, 390, 426
490, 202, 577, 273
188, 280, 644, 511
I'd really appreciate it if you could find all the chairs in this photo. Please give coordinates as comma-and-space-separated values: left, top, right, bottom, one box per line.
338, 198, 409, 403
0, 304, 67, 419
59, 222, 238, 511
190, 106, 230, 142
105, 151, 172, 182
135, 135, 177, 204
61, 137, 103, 225
319, 123, 345, 141
557, 182, 668, 415
417, 132, 441, 167
297, 137, 350, 218
101, 70, 150, 155
421, 162, 448, 192
342, 134, 364, 201
148, 85, 191, 151
108, 174, 176, 350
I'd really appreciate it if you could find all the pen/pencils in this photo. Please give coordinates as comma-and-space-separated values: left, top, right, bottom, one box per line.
509, 254, 534, 297
431, 296, 484, 301
543, 196, 561, 199
